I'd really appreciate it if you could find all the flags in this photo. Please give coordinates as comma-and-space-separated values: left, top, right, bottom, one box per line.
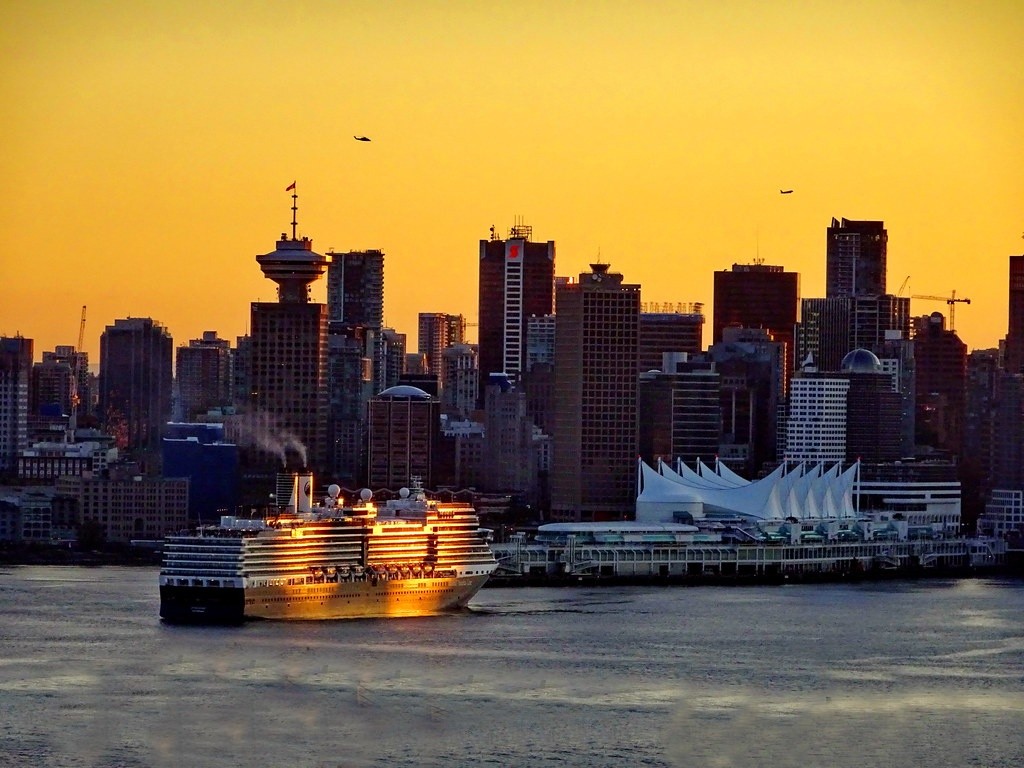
286, 183, 295, 191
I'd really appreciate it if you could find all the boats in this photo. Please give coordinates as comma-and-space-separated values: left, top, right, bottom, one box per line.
159, 466, 500, 626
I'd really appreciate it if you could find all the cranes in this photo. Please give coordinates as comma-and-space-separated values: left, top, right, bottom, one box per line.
911, 290, 970, 334
64, 306, 86, 443
